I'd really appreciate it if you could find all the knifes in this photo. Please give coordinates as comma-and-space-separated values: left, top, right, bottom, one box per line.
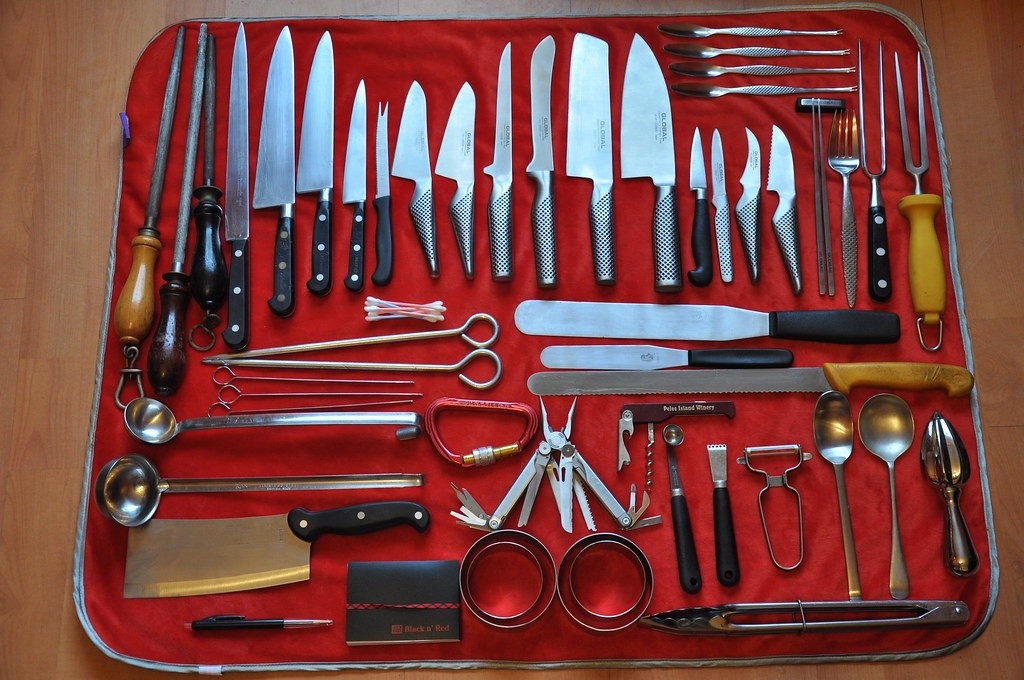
538, 346, 795, 370
735, 126, 762, 285
371, 101, 392, 284
123, 502, 430, 599
343, 78, 366, 295
482, 41, 515, 282
526, 362, 973, 395
252, 24, 304, 318
514, 298, 901, 345
687, 126, 714, 288
711, 128, 735, 283
525, 32, 557, 290
220, 19, 250, 354
620, 32, 682, 291
433, 81, 476, 281
390, 79, 440, 281
565, 33, 614, 286
296, 30, 333, 299
766, 124, 803, 297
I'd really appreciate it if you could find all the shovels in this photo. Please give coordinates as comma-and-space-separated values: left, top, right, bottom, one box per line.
920, 411, 981, 578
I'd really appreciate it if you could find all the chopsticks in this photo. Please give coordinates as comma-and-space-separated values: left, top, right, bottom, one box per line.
812, 97, 835, 296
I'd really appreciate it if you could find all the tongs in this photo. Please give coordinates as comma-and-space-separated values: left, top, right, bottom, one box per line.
638, 599, 972, 635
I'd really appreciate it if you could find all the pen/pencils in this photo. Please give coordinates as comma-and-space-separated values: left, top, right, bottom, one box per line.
188, 614, 334, 630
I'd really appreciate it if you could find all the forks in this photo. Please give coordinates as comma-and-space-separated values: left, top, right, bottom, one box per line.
828, 109, 859, 309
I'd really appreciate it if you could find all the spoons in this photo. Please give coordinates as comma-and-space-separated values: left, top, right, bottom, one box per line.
858, 392, 915, 601
813, 390, 864, 603
